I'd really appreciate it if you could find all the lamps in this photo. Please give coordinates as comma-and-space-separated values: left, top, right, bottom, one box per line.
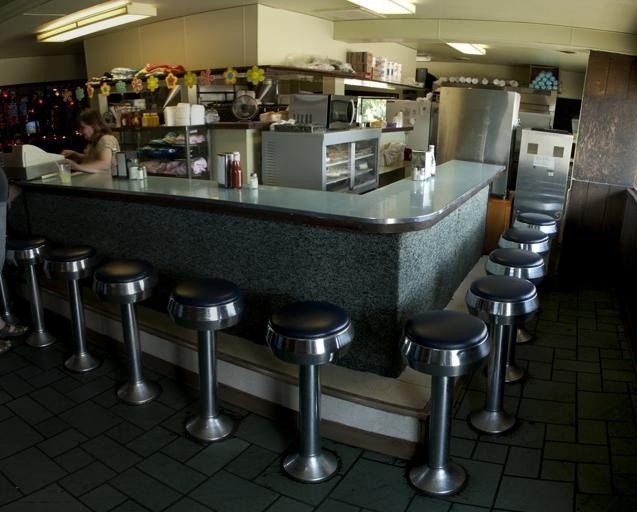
348, 0, 416, 16
32, 1, 157, 44
446, 42, 486, 56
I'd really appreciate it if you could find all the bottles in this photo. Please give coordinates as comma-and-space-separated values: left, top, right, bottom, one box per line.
429, 145, 437, 175
411, 167, 425, 181
227, 154, 234, 188
234, 151, 242, 189
110, 179, 147, 191
128, 158, 147, 180
362, 114, 370, 127
380, 111, 403, 128
111, 148, 118, 176
249, 189, 258, 205
372, 56, 401, 81
120, 107, 160, 128
413, 176, 435, 195
249, 173, 259, 189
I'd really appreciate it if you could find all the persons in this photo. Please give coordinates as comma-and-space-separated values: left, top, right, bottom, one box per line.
58, 106, 122, 175
0, 168, 31, 356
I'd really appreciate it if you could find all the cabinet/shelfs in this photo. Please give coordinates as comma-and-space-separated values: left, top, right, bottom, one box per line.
356, 97, 414, 187
263, 130, 379, 194
103, 126, 209, 179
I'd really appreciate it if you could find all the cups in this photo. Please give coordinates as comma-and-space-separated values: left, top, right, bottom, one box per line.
56, 159, 72, 183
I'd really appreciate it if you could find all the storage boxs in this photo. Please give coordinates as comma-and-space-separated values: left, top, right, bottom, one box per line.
346, 52, 402, 84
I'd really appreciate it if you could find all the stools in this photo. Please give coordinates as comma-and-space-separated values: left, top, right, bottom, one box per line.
43, 245, 101, 372
265, 300, 355, 483
465, 210, 560, 439
169, 276, 243, 442
93, 258, 163, 407
1, 273, 22, 326
5, 237, 57, 349
401, 311, 492, 496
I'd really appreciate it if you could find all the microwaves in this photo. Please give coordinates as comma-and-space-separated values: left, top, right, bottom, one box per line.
289, 94, 361, 131
